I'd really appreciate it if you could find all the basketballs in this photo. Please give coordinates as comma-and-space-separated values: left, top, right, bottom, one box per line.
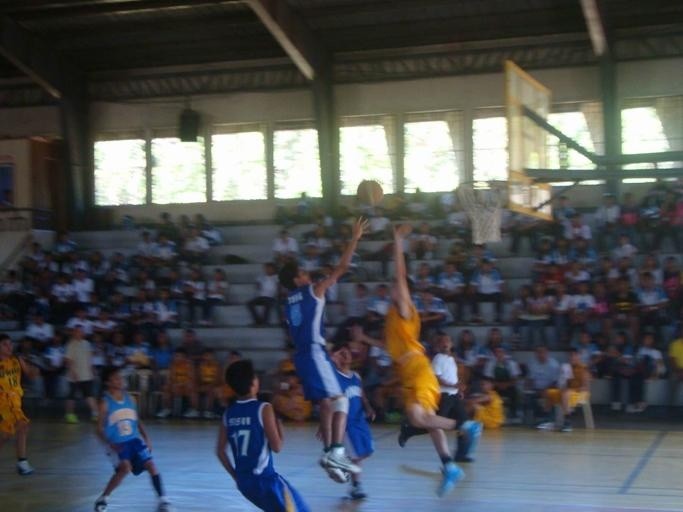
357, 179, 382, 207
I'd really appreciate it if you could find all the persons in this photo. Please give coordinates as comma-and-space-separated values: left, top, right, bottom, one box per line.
280, 215, 373, 483
0, 334, 39, 474
329, 342, 379, 501
1, 210, 236, 424
398, 330, 477, 463
381, 222, 482, 501
93, 366, 172, 511
215, 359, 307, 512
246, 179, 683, 432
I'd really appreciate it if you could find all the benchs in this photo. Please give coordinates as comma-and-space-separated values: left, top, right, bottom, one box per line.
0, 212, 683, 417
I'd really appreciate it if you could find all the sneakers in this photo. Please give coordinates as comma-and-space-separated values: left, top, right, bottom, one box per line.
437, 467, 464, 496
399, 421, 409, 447
65, 414, 79, 425
456, 419, 481, 462
318, 445, 367, 501
15, 461, 35, 475
94, 496, 109, 512
158, 501, 171, 512
155, 407, 220, 419
561, 425, 574, 432
